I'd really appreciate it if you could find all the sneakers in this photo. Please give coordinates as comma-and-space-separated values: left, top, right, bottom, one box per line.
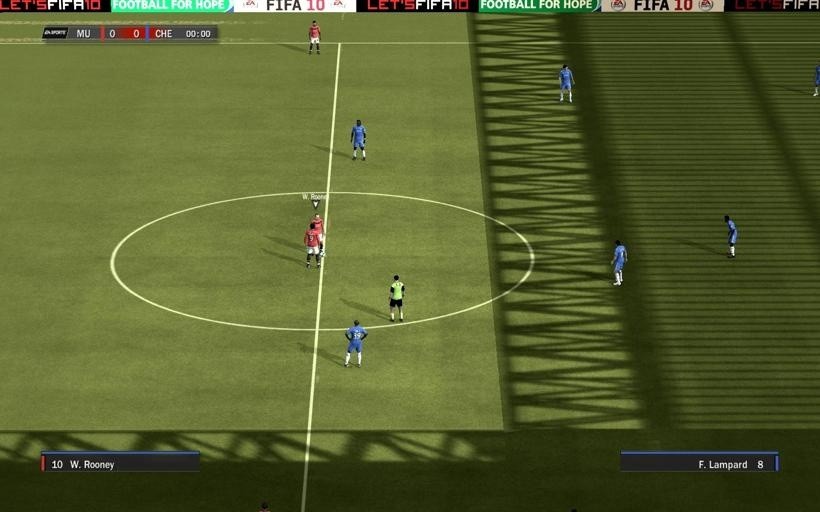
363, 157, 366, 161
727, 252, 736, 258
358, 364, 361, 368
613, 278, 623, 286
390, 318, 394, 322
344, 363, 349, 367
307, 264, 311, 268
560, 98, 572, 103
353, 157, 357, 160
317, 264, 320, 268
399, 318, 404, 322
